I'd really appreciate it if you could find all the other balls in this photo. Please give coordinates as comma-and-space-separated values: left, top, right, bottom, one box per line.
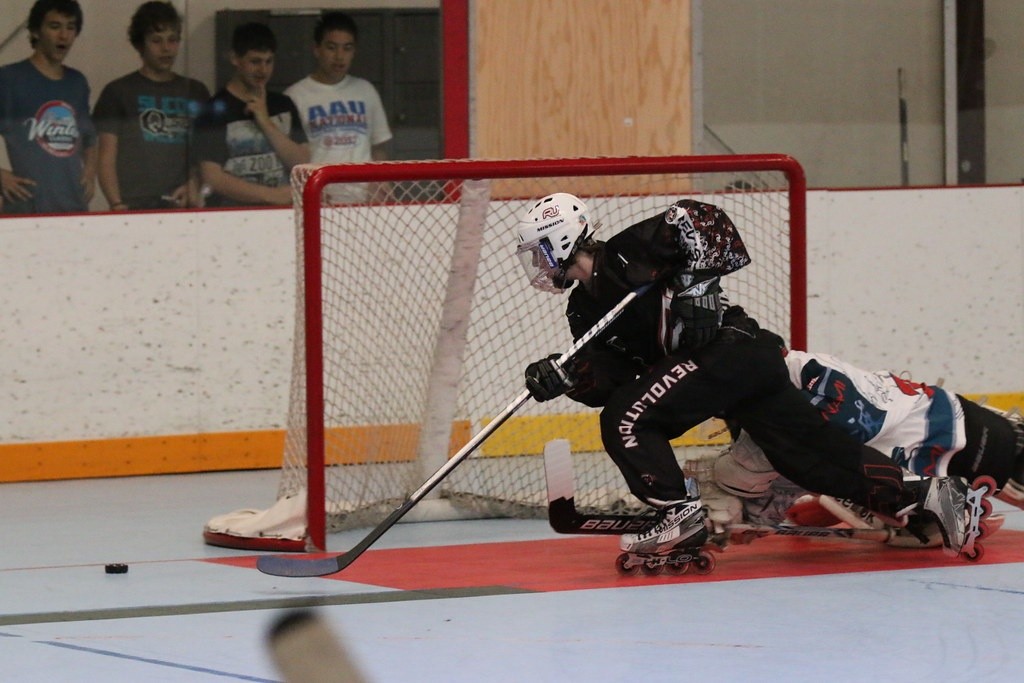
104, 562, 129, 575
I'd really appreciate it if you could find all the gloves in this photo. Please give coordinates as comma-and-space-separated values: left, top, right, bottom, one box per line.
524, 353, 579, 402
666, 268, 728, 356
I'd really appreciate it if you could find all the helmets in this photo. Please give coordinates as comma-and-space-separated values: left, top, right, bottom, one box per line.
515, 193, 597, 290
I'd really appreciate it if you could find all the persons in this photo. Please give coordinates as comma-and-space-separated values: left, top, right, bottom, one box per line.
91, 0, 211, 209
193, 21, 331, 207
0, 0, 96, 213
284, 12, 395, 203
515, 192, 996, 575
684, 328, 1024, 546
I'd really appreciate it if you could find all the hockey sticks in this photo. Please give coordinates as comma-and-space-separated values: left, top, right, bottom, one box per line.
255, 276, 894, 579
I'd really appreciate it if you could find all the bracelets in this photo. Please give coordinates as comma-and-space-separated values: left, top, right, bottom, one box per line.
111, 202, 123, 208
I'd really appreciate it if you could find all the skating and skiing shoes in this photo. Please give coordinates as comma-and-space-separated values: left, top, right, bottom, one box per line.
615, 495, 716, 576
895, 476, 996, 561
